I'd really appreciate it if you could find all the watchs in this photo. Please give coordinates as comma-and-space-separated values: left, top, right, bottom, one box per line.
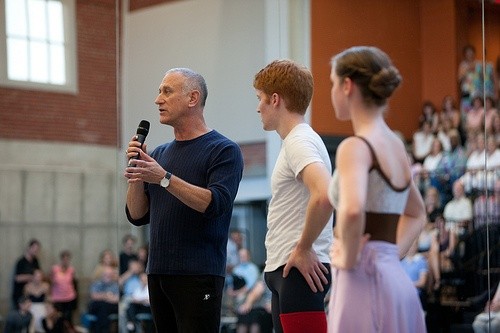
160, 171, 172, 189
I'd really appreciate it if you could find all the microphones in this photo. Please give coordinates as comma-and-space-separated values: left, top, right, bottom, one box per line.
128, 120, 150, 178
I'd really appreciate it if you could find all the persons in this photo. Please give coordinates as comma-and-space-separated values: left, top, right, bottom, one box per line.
400, 45, 500, 333
125, 67, 244, 333
11, 230, 274, 333
252, 59, 332, 333
326, 44, 428, 333
